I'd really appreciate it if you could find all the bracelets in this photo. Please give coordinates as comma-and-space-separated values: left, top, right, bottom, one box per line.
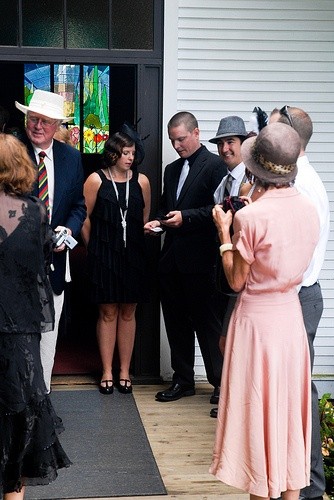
220, 244, 233, 256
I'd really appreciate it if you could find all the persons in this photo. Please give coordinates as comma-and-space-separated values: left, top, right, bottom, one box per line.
213, 104, 328, 500
13, 89, 88, 392
81, 134, 161, 394
141, 111, 229, 402
209, 122, 320, 500
0, 133, 72, 500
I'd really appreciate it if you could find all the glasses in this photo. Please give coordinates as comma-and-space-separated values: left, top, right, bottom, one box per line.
281, 105, 293, 127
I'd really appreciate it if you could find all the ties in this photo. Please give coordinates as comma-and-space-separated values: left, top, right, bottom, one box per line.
37, 151, 50, 219
176, 160, 190, 202
223, 174, 235, 199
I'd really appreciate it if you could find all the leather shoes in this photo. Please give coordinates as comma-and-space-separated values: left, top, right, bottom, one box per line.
156, 381, 197, 402
210, 388, 221, 404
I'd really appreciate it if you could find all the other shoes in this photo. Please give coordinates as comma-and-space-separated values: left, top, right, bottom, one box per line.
119, 378, 133, 394
100, 379, 115, 395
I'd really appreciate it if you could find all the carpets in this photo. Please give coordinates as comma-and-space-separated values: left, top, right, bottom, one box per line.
0, 388, 168, 500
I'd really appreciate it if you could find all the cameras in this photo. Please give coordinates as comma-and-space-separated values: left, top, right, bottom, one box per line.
50, 229, 68, 249
222, 196, 246, 214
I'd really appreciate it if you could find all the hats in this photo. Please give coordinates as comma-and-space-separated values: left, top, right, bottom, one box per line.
14, 89, 76, 124
207, 116, 251, 144
240, 122, 301, 183
